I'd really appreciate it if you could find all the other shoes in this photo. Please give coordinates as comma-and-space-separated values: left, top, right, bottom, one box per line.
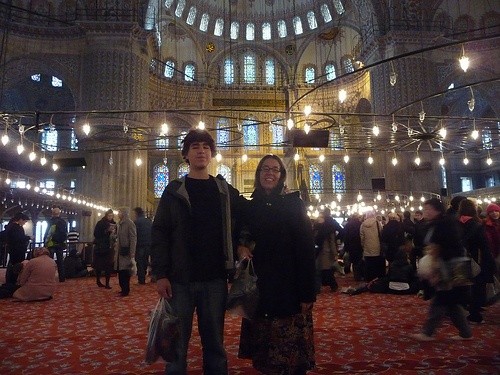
464, 313, 484, 324
409, 332, 472, 343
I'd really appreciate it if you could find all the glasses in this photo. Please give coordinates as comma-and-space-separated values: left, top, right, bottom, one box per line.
260, 166, 281, 173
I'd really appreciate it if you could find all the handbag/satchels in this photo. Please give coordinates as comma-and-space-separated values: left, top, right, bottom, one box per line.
230, 258, 259, 302
143, 297, 182, 364
120, 246, 130, 256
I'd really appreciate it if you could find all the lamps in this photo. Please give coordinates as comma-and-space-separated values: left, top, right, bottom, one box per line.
2, 124, 119, 215
287, 57, 492, 166
307, 192, 425, 224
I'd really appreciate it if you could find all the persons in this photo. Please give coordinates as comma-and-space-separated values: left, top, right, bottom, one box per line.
0, 214, 33, 297
150, 129, 249, 375
311, 200, 409, 294
409, 195, 500, 342
235, 154, 319, 375
43, 208, 68, 282
62, 226, 96, 279
14, 247, 59, 302
114, 206, 137, 297
132, 206, 153, 284
92, 209, 118, 289
